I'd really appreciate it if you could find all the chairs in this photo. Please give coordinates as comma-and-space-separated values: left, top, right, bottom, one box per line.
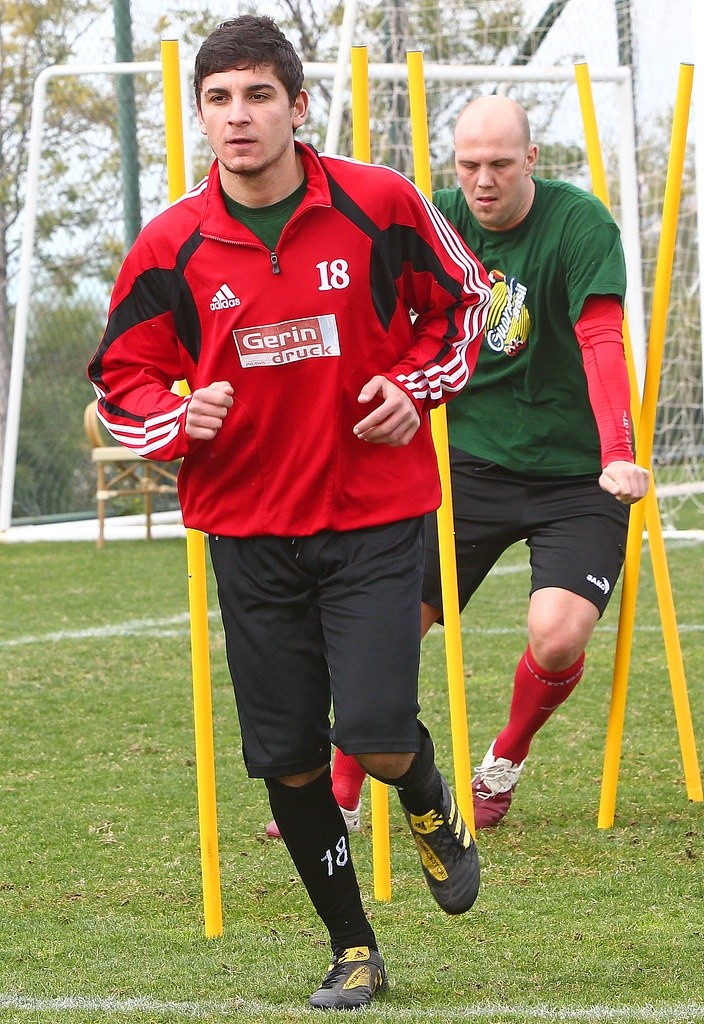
82, 399, 186, 548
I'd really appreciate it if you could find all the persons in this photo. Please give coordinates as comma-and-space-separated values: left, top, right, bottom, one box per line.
260, 93, 651, 840
84, 9, 494, 1014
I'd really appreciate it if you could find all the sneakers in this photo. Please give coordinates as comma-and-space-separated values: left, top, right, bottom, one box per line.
399, 772, 481, 915
311, 944, 386, 1010
473, 738, 527, 829
266, 798, 362, 839
